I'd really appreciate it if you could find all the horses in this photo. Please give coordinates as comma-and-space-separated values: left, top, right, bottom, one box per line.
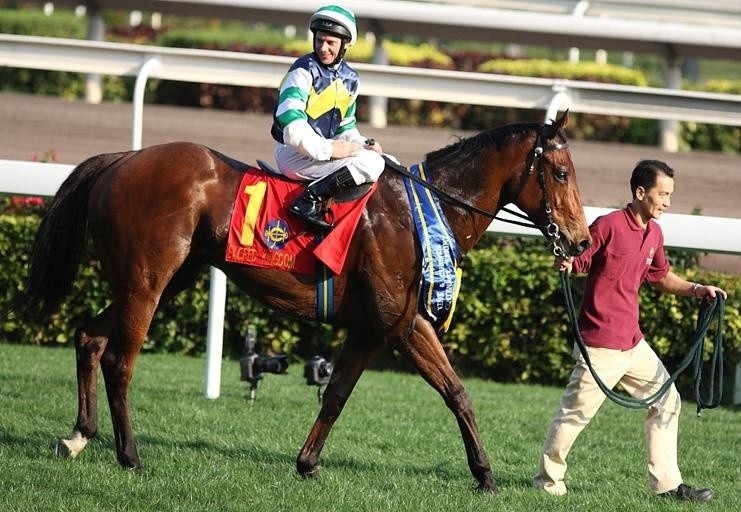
0, 107, 592, 493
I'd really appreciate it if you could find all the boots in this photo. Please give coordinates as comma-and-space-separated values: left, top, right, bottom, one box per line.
289, 166, 356, 227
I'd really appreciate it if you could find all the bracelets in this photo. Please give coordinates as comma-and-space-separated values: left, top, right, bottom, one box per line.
690, 283, 700, 296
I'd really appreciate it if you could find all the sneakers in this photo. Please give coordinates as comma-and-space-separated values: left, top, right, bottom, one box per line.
658, 484, 714, 501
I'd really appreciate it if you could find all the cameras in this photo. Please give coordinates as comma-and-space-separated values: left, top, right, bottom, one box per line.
240, 327, 289, 385
303, 354, 334, 386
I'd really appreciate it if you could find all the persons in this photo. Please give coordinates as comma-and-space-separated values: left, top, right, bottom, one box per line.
271, 4, 400, 229
530, 160, 728, 502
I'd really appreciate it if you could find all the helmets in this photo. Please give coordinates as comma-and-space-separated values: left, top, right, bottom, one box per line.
309, 5, 357, 49
